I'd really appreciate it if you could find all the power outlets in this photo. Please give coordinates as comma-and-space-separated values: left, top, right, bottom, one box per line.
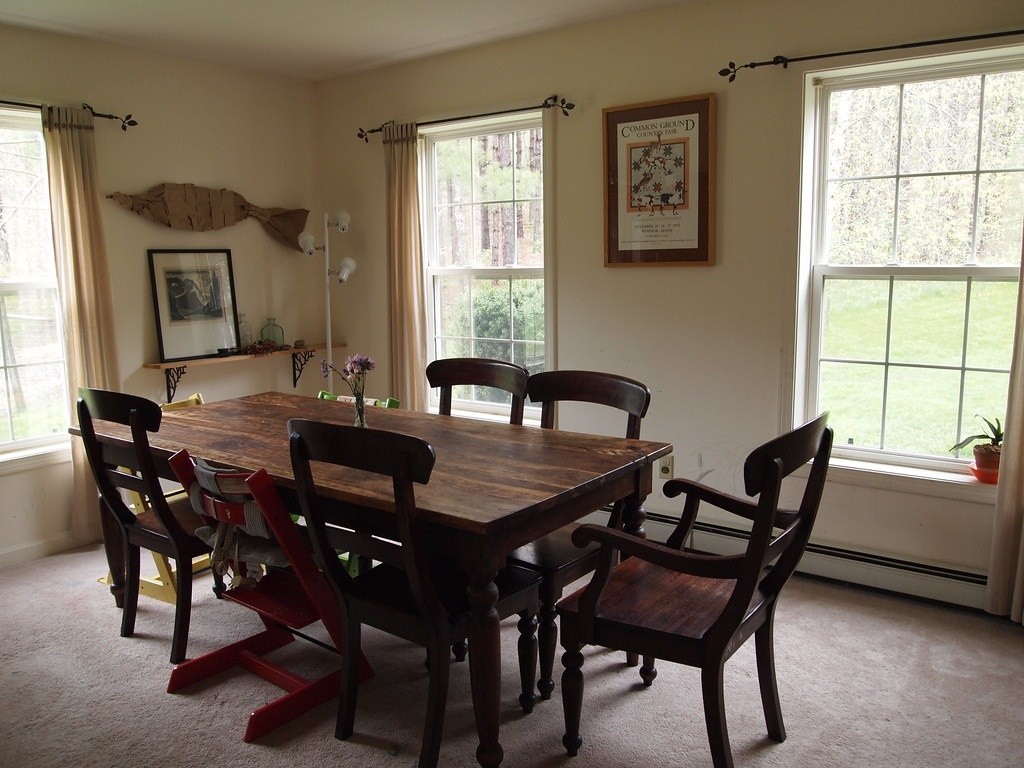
658, 455, 674, 480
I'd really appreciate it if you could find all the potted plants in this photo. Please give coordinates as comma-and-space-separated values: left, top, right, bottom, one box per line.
948, 414, 1004, 470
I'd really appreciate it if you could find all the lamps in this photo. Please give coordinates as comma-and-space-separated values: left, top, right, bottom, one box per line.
297, 212, 351, 394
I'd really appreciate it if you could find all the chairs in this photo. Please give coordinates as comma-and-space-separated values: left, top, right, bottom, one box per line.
166, 448, 380, 744
507, 370, 653, 701
555, 411, 833, 768
289, 390, 401, 577
285, 414, 544, 768
76, 385, 212, 665
97, 392, 212, 604
426, 357, 530, 426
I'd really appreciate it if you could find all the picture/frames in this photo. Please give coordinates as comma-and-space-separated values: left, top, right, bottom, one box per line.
146, 248, 243, 363
602, 91, 717, 269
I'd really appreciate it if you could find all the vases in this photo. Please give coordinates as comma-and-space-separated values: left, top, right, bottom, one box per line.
352, 394, 369, 428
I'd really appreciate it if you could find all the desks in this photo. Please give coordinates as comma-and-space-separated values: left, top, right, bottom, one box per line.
66, 392, 675, 768
142, 342, 347, 404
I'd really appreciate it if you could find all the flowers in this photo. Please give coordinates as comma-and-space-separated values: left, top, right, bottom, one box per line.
319, 353, 377, 428
